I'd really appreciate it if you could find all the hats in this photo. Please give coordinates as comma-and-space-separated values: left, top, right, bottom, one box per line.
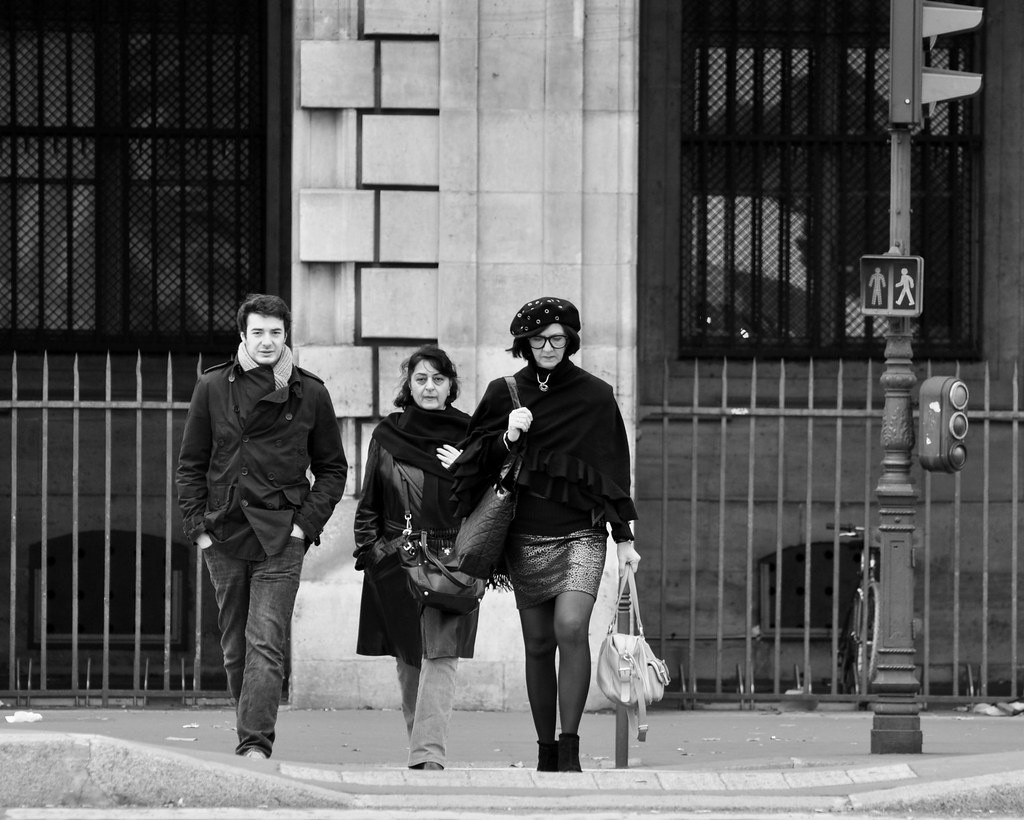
510, 297, 581, 338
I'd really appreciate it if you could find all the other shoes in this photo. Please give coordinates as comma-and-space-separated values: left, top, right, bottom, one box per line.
424, 762, 444, 771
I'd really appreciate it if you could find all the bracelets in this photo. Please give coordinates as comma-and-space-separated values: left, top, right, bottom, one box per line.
616, 539, 632, 544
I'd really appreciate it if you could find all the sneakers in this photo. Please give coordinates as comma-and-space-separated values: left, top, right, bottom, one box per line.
243, 748, 268, 759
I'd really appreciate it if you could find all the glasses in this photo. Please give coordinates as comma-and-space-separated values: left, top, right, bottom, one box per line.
528, 333, 572, 349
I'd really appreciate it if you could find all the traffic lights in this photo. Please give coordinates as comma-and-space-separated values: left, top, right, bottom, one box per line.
919, 377, 970, 474
889, 1, 984, 132
860, 255, 925, 316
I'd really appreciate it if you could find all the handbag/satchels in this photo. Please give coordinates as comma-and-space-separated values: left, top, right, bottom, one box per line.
454, 374, 528, 583
379, 452, 486, 615
596, 563, 671, 742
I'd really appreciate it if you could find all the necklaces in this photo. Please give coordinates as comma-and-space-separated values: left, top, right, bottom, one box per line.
536, 373, 550, 392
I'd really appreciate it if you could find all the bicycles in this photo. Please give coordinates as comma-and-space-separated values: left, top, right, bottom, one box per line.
826, 522, 881, 703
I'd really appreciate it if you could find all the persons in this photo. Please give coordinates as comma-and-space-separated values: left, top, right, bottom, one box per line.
451, 298, 641, 772
175, 293, 347, 762
353, 348, 480, 769
